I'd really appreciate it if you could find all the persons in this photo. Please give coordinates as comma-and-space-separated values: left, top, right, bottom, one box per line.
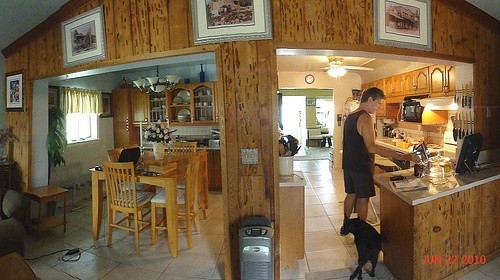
340, 87, 415, 235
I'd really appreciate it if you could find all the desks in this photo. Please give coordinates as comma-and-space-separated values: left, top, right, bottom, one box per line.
0, 165, 20, 220
89, 157, 194, 259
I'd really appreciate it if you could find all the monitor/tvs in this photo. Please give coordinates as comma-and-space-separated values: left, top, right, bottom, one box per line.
454, 132, 484, 174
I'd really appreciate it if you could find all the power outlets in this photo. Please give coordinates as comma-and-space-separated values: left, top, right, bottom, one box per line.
242, 148, 259, 164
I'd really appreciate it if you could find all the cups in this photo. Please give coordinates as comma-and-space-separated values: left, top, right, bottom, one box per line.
195, 91, 213, 106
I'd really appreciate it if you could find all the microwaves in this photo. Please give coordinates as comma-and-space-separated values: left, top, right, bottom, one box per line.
403, 101, 423, 123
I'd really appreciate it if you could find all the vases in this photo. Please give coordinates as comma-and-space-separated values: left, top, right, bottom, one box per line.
278, 155, 294, 176
152, 141, 164, 161
0, 142, 8, 164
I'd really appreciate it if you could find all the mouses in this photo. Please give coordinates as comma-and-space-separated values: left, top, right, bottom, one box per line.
95, 167, 102, 171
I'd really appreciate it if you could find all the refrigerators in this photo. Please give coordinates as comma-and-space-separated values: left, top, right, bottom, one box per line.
343, 100, 376, 127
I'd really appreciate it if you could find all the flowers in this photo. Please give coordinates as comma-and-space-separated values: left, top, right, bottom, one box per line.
144, 119, 178, 145
0, 126, 21, 145
278, 135, 301, 157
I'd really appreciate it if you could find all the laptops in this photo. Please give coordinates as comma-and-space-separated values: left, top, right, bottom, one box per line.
107, 147, 140, 170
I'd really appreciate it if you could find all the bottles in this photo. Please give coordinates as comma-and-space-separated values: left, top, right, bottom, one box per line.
413, 163, 420, 176
199, 64, 205, 82
382, 123, 394, 138
167, 147, 172, 163
428, 152, 448, 183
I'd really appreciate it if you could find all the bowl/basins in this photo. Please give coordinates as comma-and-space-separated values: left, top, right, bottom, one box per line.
178, 115, 187, 122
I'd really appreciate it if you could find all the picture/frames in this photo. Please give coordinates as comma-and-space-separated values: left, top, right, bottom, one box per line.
5, 5, 113, 119
306, 98, 316, 106
190, 0, 273, 46
373, 0, 432, 52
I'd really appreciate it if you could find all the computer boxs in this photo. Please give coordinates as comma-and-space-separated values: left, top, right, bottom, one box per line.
238, 215, 275, 279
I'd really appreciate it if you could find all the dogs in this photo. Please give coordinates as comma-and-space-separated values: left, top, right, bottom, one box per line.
340, 212, 383, 280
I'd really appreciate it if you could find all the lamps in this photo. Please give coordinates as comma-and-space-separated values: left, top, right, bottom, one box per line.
132, 66, 180, 95
327, 68, 347, 87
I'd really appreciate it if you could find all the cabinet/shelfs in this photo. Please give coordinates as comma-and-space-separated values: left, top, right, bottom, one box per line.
142, 150, 222, 193
361, 64, 456, 119
280, 170, 305, 270
114, 81, 216, 148
27, 185, 68, 241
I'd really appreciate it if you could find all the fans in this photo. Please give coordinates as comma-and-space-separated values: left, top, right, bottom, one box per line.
319, 55, 375, 72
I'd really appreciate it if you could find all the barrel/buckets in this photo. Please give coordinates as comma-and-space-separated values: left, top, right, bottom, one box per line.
279, 156, 292, 175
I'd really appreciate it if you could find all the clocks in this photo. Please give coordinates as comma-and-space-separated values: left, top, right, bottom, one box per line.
305, 74, 315, 84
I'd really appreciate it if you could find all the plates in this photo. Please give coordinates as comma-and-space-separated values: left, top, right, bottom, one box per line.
173, 90, 191, 115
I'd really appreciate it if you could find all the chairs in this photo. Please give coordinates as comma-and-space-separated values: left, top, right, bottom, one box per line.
102, 142, 209, 255
307, 127, 333, 148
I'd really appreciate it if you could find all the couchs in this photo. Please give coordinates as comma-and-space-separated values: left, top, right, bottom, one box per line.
0, 189, 24, 257
